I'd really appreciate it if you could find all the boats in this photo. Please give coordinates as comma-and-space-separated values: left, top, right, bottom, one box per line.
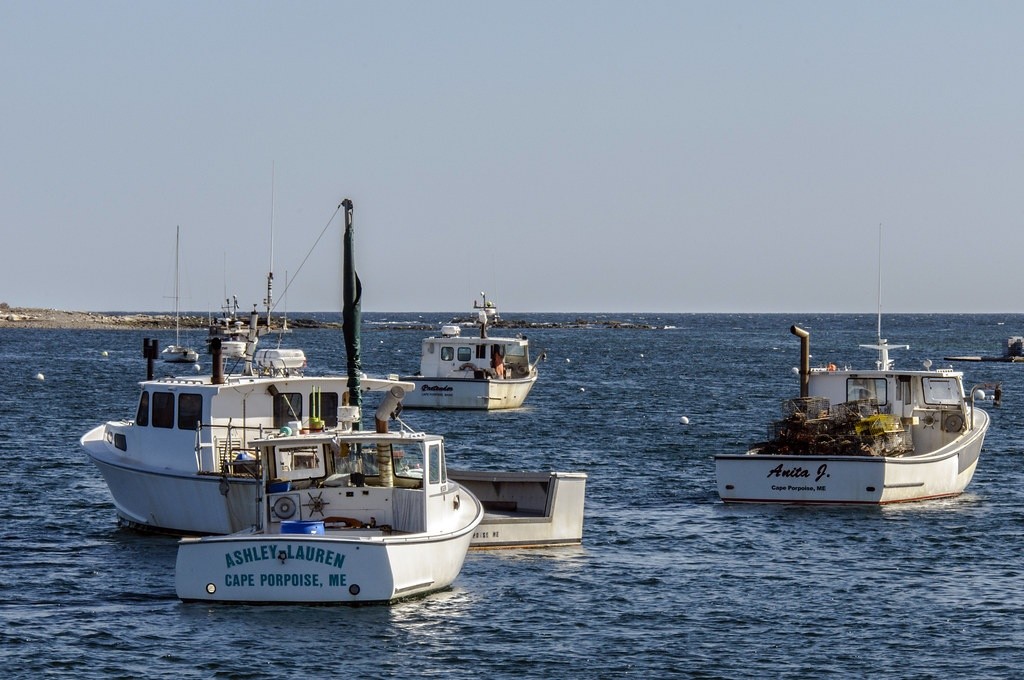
715, 225, 990, 507
172, 388, 485, 607
390, 292, 547, 410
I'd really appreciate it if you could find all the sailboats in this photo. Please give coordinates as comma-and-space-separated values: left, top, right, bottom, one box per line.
159, 161, 307, 370
79, 196, 589, 548
